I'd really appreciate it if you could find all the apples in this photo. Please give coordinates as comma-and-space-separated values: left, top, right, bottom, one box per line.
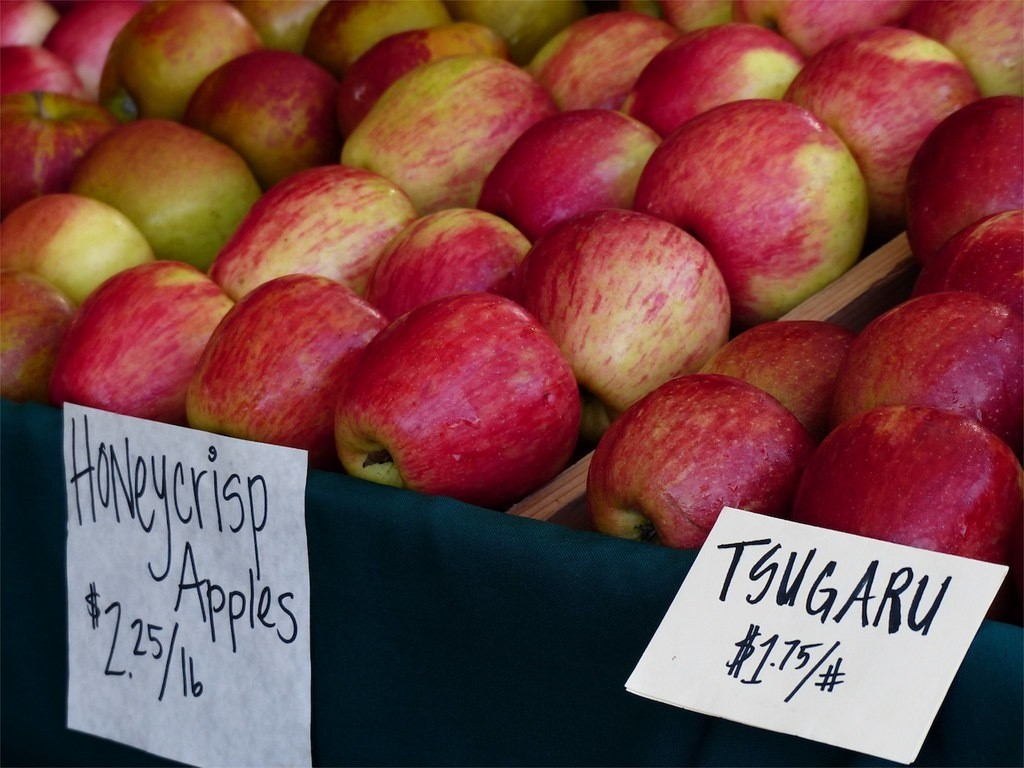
0, 0, 1024, 618
